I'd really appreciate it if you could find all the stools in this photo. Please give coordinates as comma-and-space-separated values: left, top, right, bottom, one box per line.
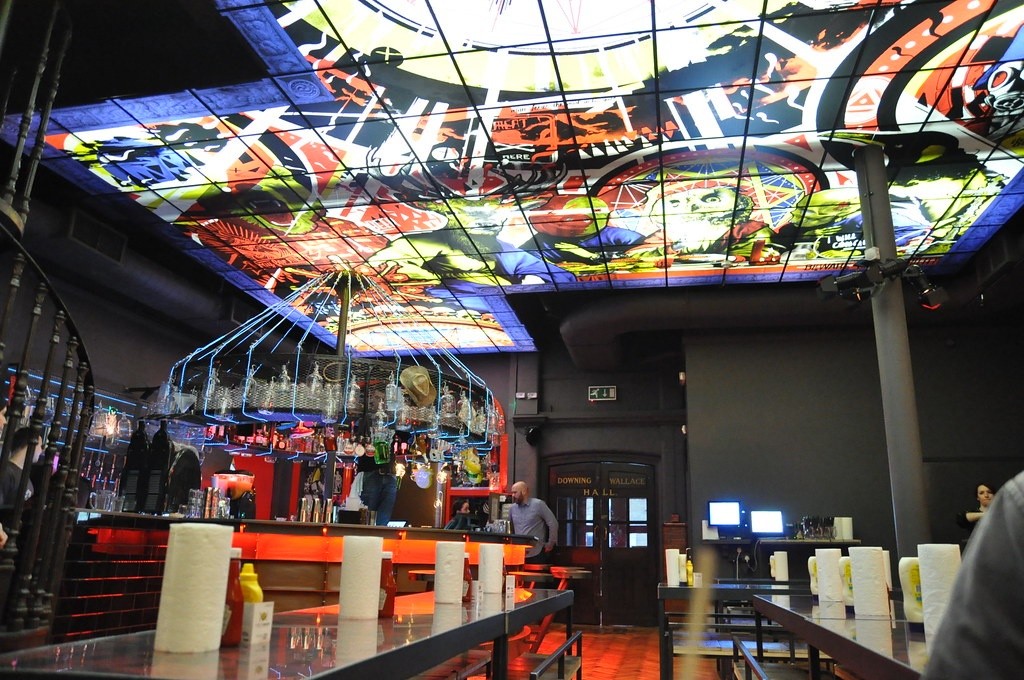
732, 638, 843, 680
507, 631, 584, 680
723, 605, 780, 674
406, 567, 592, 652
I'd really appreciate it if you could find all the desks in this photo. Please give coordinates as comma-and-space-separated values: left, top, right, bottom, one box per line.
656, 583, 931, 680
1, 589, 573, 680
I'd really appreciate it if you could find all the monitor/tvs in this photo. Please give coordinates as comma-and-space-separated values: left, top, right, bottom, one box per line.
708, 500, 745, 527
748, 509, 786, 535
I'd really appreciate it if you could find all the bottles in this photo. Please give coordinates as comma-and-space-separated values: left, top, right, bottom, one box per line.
158, 362, 500, 435
770, 555, 776, 579
378, 551, 397, 618
300, 497, 332, 522
898, 557, 924, 631
793, 514, 836, 538
221, 548, 264, 645
679, 554, 694, 586
839, 556, 854, 612
462, 552, 473, 603
23, 385, 132, 440
502, 552, 507, 593
808, 556, 818, 602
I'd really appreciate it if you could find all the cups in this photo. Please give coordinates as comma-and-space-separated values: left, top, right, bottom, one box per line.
90, 489, 126, 513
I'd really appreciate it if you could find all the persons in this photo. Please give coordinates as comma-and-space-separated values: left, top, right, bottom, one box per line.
506, 480, 558, 563
954, 482, 998, 533
445, 499, 470, 529
0, 427, 42, 506
922, 470, 1024, 680
357, 453, 397, 525
0, 397, 9, 549
